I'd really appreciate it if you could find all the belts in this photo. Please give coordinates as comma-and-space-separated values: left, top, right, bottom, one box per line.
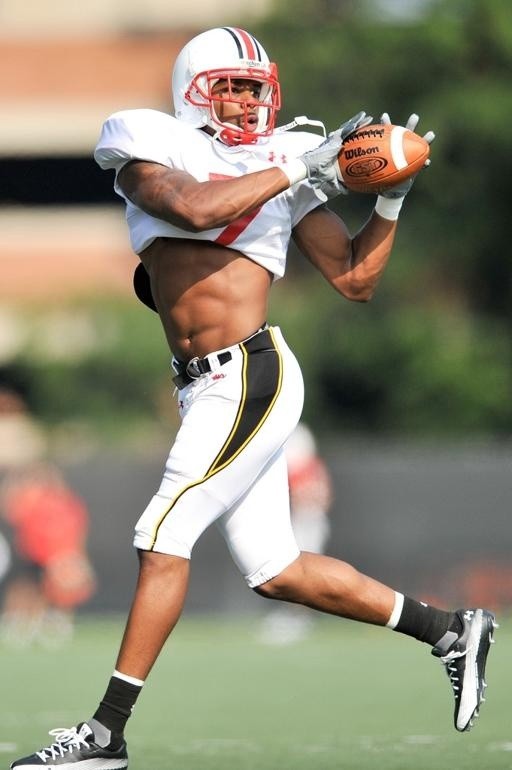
171, 331, 275, 391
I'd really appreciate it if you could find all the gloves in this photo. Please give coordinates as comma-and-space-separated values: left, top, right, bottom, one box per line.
297, 112, 374, 179
379, 112, 436, 199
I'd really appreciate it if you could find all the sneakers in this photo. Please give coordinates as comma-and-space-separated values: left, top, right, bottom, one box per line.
431, 608, 499, 733
9, 717, 129, 770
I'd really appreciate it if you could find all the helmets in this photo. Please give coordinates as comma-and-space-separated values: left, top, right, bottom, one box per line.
172, 26, 282, 146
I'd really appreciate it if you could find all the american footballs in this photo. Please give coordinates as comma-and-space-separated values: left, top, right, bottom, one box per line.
333, 125, 430, 192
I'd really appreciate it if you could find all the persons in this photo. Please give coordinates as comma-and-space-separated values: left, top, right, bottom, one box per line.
264, 419, 331, 643
1, 361, 95, 643
9, 25, 500, 770
